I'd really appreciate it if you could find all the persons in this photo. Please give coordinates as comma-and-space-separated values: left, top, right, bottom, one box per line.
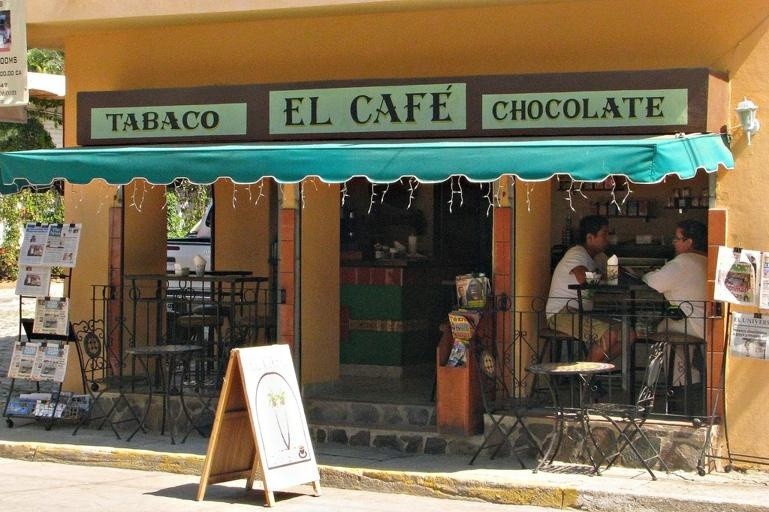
0, 15, 10, 45
640, 219, 709, 384
542, 214, 624, 405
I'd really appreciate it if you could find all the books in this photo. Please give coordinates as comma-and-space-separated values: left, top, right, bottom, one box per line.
448, 273, 489, 368
40, 224, 84, 269
32, 297, 70, 335
8, 391, 92, 422
15, 263, 50, 298
8, 341, 41, 380
16, 223, 50, 266
31, 342, 70, 382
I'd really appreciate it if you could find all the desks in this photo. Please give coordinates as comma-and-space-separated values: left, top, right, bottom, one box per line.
121, 343, 207, 446
565, 284, 655, 406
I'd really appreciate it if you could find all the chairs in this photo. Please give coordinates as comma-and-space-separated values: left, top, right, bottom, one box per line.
69, 318, 147, 440
172, 324, 274, 445
462, 333, 670, 480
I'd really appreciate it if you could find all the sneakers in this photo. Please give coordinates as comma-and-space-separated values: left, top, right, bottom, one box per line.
575, 375, 607, 398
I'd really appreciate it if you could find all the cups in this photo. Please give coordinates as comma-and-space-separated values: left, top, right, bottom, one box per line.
195, 264, 205, 277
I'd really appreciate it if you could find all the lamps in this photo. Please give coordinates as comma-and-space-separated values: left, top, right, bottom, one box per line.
735, 95, 760, 145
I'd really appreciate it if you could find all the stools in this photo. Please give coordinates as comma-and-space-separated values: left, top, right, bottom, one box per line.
174, 304, 275, 394
648, 331, 705, 419
530, 327, 585, 398
